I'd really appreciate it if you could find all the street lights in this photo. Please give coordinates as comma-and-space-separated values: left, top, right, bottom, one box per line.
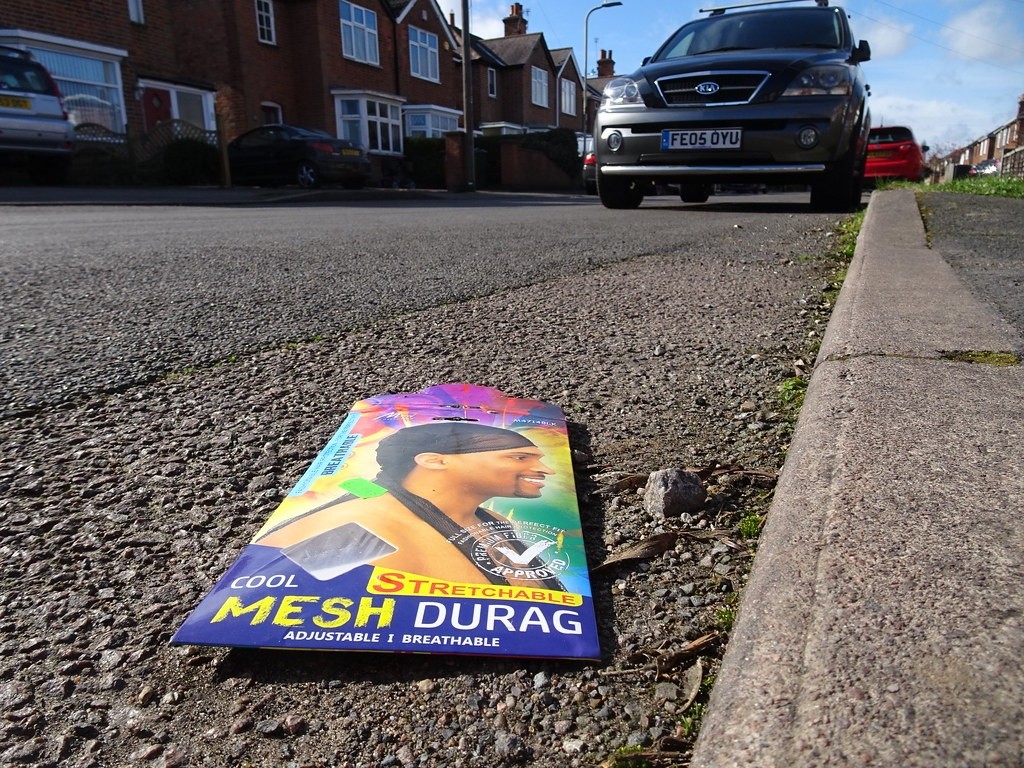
582, 1, 623, 154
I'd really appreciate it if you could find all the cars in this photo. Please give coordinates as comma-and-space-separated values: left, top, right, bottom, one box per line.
0, 43, 80, 186
950, 157, 1002, 182
863, 124, 929, 187
227, 122, 366, 192
573, 134, 598, 197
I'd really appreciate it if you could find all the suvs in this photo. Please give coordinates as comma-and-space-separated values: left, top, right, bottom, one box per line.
592, 1, 873, 213
